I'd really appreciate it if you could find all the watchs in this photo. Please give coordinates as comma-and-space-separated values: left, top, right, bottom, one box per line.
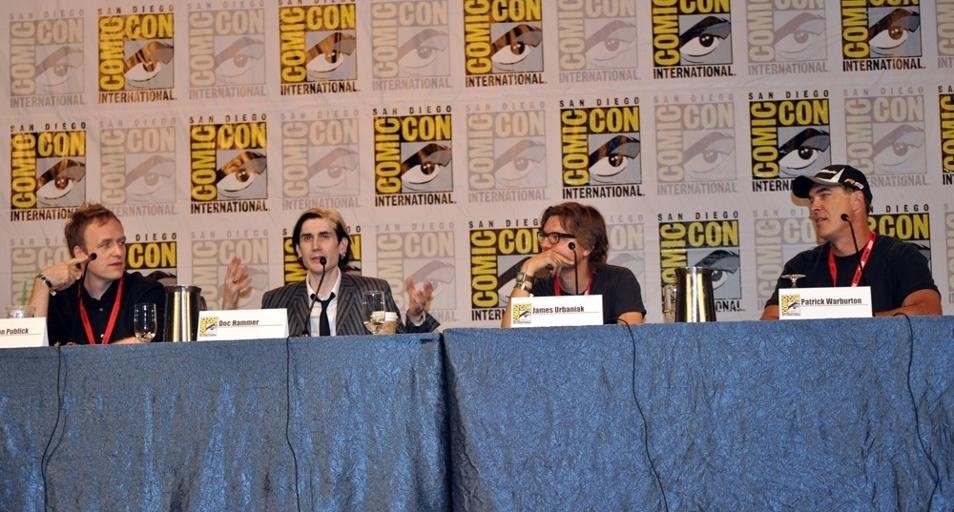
516, 271, 537, 284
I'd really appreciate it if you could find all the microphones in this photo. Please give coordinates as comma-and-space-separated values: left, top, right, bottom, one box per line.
841, 213, 866, 285
568, 241, 578, 295
301, 255, 327, 337
64, 253, 97, 344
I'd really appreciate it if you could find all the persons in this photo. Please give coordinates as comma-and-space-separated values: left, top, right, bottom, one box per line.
500, 199, 646, 328
24, 204, 168, 349
220, 206, 441, 336
757, 163, 943, 322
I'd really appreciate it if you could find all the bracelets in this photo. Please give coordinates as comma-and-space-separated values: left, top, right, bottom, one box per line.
32, 272, 57, 290
512, 283, 532, 294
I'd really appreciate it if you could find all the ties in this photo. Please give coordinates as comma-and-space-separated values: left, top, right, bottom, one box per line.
309, 291, 337, 338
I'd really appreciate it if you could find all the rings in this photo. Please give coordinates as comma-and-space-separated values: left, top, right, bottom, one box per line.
232, 278, 239, 282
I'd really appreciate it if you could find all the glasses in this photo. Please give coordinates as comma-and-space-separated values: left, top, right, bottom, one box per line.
535, 230, 576, 245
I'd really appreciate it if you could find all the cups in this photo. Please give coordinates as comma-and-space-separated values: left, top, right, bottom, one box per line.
132, 303, 157, 343
372, 310, 397, 334
362, 291, 385, 334
5, 304, 35, 318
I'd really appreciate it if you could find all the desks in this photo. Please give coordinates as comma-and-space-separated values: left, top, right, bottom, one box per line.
1, 313, 949, 511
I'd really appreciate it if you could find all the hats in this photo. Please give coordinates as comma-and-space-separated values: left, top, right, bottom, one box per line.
790, 164, 873, 203
